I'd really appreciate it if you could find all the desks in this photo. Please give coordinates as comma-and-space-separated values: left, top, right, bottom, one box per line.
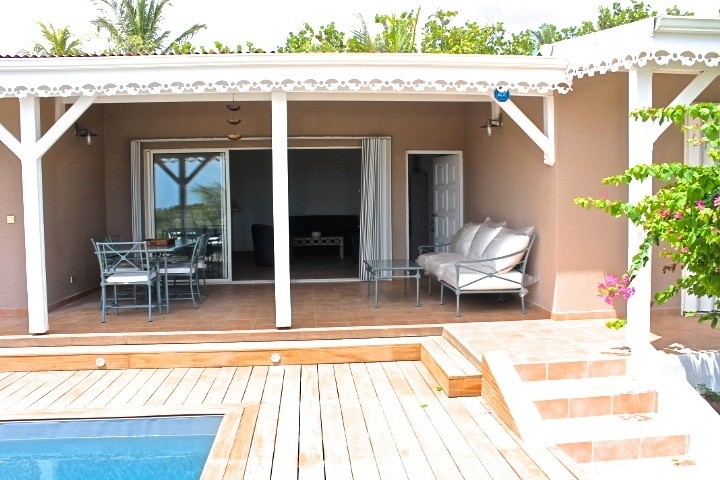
94, 239, 197, 313
292, 236, 344, 260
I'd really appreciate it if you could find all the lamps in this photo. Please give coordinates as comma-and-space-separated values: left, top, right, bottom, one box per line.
479, 114, 503, 136
74, 122, 97, 145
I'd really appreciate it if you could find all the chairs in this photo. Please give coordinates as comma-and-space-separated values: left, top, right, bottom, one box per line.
91, 231, 211, 323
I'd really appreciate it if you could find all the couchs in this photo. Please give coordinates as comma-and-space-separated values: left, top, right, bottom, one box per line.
251, 215, 359, 267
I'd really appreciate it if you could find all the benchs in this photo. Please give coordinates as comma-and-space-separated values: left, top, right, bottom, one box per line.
418, 220, 535, 316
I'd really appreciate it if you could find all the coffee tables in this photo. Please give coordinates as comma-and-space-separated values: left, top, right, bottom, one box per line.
364, 258, 424, 308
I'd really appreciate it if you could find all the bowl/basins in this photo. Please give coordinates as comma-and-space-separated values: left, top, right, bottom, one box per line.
311, 231, 321, 238
